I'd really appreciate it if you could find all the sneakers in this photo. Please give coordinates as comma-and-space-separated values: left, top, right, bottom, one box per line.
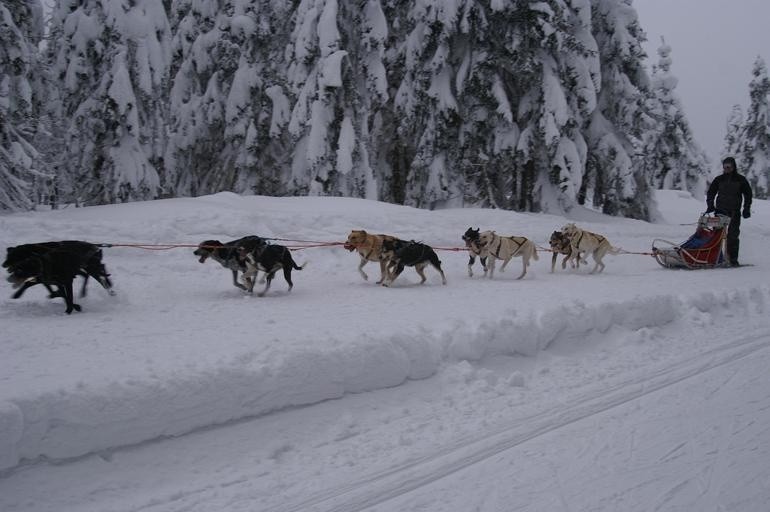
731, 261, 739, 267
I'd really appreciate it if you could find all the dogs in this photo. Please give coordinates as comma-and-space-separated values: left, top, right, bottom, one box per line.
479, 229, 539, 280
379, 238, 448, 287
193, 235, 267, 295
549, 230, 587, 273
461, 227, 488, 278
1, 239, 117, 316
344, 230, 398, 285
236, 237, 308, 298
560, 222, 623, 275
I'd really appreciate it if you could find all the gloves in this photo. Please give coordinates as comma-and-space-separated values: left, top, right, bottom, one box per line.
743, 210, 750, 218
708, 206, 715, 212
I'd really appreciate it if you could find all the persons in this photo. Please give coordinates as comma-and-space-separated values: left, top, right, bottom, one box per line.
706, 156, 753, 267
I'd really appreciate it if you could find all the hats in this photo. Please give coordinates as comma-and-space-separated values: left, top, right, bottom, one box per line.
724, 157, 735, 166
